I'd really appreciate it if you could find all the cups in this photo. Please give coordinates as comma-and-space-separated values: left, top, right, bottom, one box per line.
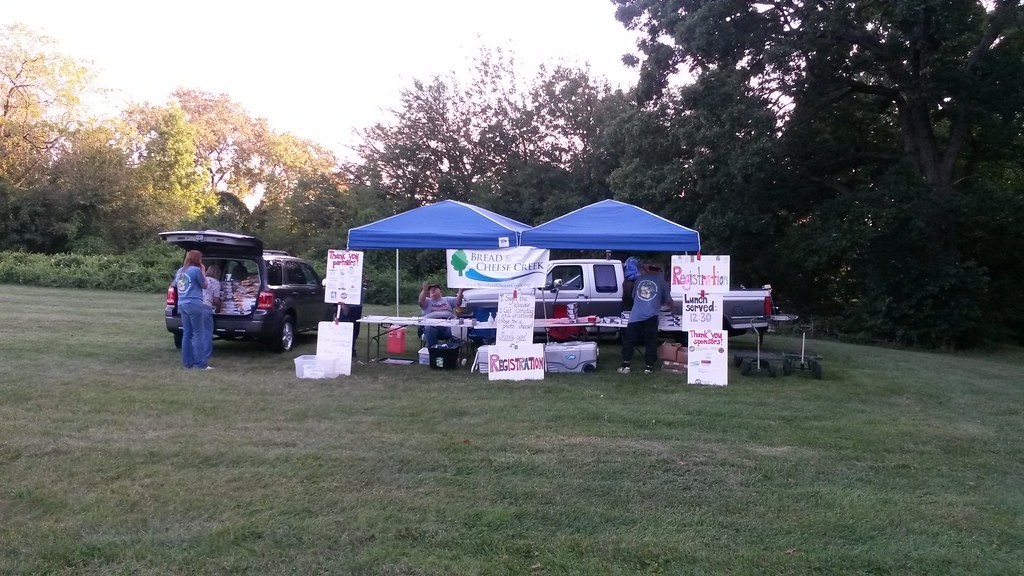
459, 318, 464, 324
472, 318, 477, 325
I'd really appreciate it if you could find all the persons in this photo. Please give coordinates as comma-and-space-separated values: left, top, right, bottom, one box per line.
418, 282, 465, 348
616, 261, 675, 375
175, 250, 215, 371
340, 274, 368, 357
201, 264, 220, 359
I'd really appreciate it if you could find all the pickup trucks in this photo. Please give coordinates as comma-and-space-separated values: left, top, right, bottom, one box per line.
456, 258, 799, 345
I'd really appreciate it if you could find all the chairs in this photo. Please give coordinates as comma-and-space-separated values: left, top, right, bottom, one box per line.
545, 304, 589, 343
472, 306, 498, 342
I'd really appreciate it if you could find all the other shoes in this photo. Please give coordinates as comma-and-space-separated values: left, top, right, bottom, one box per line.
644, 365, 653, 374
617, 361, 630, 374
200, 366, 215, 371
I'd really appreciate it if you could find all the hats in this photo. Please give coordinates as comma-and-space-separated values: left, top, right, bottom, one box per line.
427, 284, 441, 291
649, 261, 664, 272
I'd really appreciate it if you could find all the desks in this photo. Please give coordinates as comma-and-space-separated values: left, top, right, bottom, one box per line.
474, 319, 595, 345
356, 315, 479, 370
594, 319, 683, 359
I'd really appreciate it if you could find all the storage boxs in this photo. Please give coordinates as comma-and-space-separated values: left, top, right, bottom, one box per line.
567, 303, 579, 324
387, 326, 407, 354
477, 345, 547, 373
417, 347, 430, 365
661, 342, 688, 373
596, 306, 683, 327
427, 345, 461, 369
294, 355, 340, 379
545, 342, 599, 373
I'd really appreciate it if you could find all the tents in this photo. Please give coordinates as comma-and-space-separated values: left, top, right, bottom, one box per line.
333, 197, 533, 323
518, 198, 701, 256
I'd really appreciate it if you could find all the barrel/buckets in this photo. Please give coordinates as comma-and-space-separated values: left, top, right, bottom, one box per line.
386, 324, 406, 354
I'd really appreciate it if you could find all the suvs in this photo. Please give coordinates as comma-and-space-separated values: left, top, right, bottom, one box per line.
158, 229, 349, 353
